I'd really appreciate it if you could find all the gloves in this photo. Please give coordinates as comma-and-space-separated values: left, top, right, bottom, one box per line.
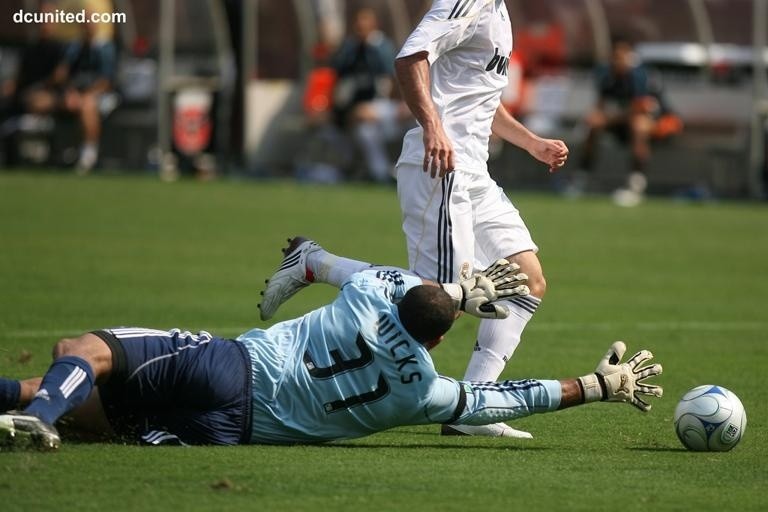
442, 259, 530, 318
581, 341, 663, 412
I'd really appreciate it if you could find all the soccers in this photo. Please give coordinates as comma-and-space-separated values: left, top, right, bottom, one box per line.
674, 384, 748, 453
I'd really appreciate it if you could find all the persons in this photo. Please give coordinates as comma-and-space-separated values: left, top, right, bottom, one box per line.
1, 1, 685, 208
256, 1, 571, 440
1, 258, 667, 452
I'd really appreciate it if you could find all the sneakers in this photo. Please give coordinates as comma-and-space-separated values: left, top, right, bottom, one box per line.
0, 412, 62, 451
442, 422, 533, 438
257, 237, 322, 320
